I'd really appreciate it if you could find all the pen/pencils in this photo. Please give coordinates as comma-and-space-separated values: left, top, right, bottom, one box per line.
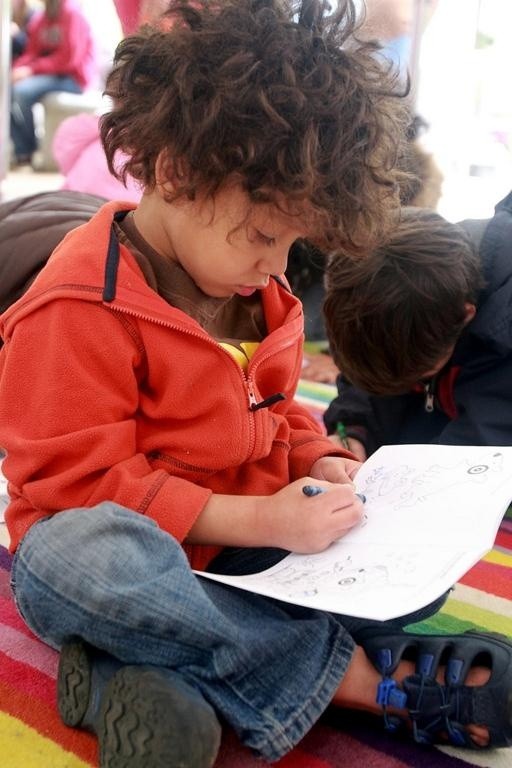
301, 484, 369, 507
336, 418, 351, 455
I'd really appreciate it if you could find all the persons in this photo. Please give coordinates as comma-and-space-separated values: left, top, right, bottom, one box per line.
56, 4, 200, 212
8, 0, 95, 173
0, 0, 512, 767
323, 193, 512, 461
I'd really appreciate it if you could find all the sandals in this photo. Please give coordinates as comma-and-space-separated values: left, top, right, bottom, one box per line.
55, 636, 221, 768
364, 629, 512, 751
